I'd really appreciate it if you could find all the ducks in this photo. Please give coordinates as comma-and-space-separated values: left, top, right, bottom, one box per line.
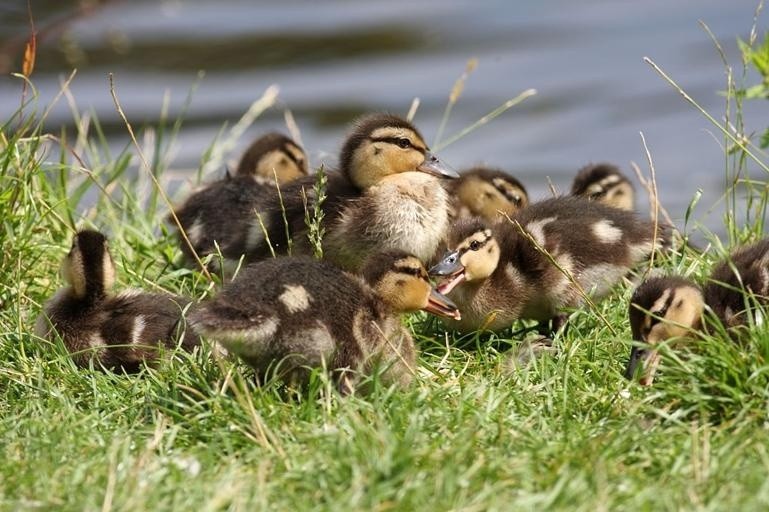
627, 239, 769, 387
33, 228, 201, 375
163, 113, 683, 402
